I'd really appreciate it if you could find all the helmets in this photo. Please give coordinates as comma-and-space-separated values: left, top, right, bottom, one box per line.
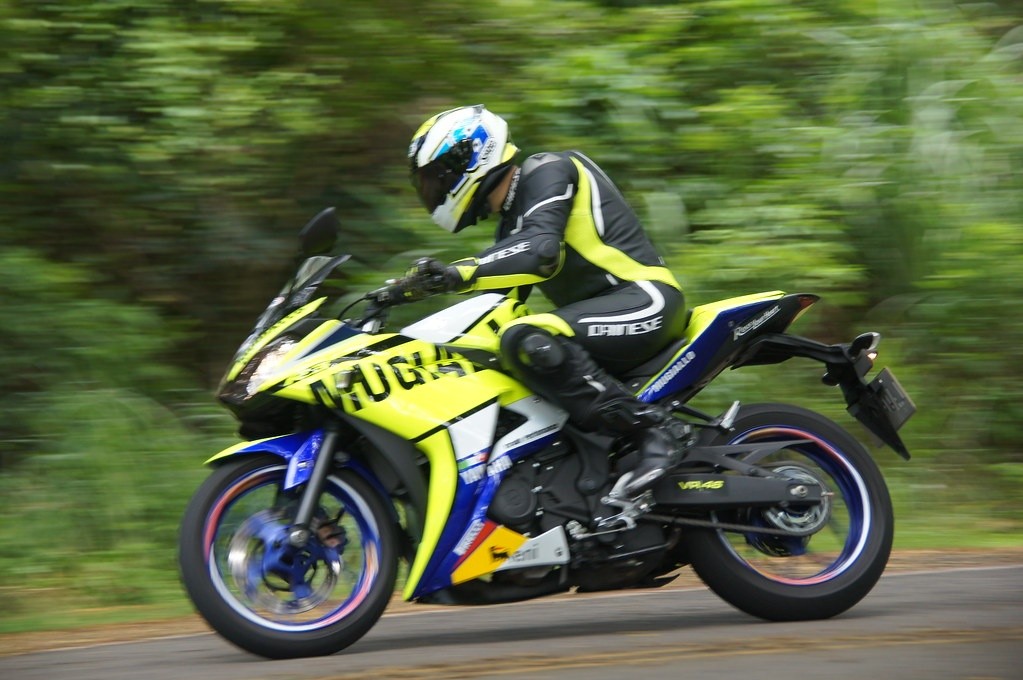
406, 103, 523, 233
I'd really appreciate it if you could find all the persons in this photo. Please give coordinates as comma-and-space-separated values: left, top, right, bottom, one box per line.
404, 104, 701, 491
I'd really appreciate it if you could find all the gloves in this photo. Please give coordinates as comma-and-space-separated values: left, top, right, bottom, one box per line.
401, 256, 460, 303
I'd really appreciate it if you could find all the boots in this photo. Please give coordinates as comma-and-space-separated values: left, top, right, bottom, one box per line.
577, 382, 702, 499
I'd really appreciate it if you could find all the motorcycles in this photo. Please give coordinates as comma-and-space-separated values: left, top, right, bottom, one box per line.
179, 205, 917, 662
